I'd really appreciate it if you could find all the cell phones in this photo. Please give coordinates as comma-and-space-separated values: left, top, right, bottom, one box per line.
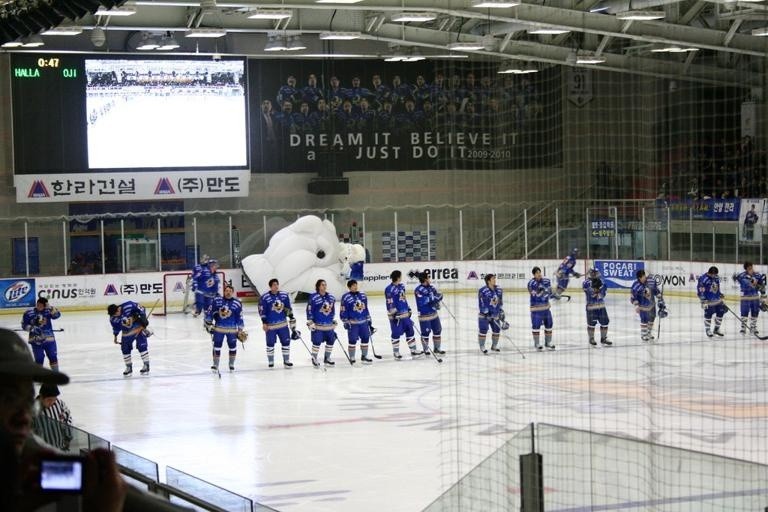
33, 454, 87, 496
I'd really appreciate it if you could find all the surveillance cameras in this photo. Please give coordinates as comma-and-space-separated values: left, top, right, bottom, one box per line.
90, 27, 106, 47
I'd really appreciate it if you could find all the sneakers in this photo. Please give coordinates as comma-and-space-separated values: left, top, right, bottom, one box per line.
554, 292, 561, 300
204, 321, 214, 332
29, 338, 45, 347
210, 365, 220, 373
193, 311, 201, 318
228, 364, 235, 372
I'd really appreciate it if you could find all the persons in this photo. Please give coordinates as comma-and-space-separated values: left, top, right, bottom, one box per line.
737, 262, 767, 335
583, 268, 613, 346
340, 280, 372, 363
596, 161, 611, 204
655, 134, 768, 201
527, 267, 555, 349
415, 272, 445, 356
306, 280, 336, 365
30, 381, 73, 450
257, 73, 544, 150
192, 254, 209, 278
22, 298, 60, 372
0, 328, 126, 512
553, 247, 581, 300
478, 275, 503, 354
204, 286, 244, 371
697, 267, 726, 338
258, 279, 296, 366
385, 271, 425, 358
631, 271, 667, 340
742, 204, 758, 241
191, 260, 219, 317
107, 301, 152, 376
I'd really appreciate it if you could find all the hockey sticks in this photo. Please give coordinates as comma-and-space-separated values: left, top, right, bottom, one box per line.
366, 322, 383, 360
118, 298, 160, 345
411, 323, 444, 363
654, 283, 665, 343
292, 325, 328, 375
553, 294, 571, 302
721, 301, 768, 341
11, 327, 66, 333
490, 318, 527, 361
210, 327, 222, 379
561, 269, 590, 280
331, 328, 356, 365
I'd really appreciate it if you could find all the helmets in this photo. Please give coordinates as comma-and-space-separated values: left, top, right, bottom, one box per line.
761, 301, 768, 311
208, 258, 218, 265
589, 266, 600, 276
573, 247, 582, 254
291, 330, 302, 340
140, 329, 153, 339
369, 326, 376, 336
657, 309, 668, 318
723, 304, 729, 313
501, 321, 510, 330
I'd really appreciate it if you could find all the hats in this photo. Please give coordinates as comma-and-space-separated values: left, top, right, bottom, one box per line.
0, 328, 70, 385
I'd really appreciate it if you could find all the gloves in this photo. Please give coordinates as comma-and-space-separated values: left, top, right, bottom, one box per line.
343, 321, 352, 330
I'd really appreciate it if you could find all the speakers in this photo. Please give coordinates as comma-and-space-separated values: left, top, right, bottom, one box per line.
317, 144, 344, 178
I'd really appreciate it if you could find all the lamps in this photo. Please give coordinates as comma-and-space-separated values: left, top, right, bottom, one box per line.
156, 34, 174, 50
264, 37, 275, 51
135, 34, 154, 50
576, 55, 606, 64
245, 10, 292, 19
271, 34, 286, 50
496, 63, 538, 74
144, 33, 160, 48
1, 32, 23, 48
90, 22, 106, 47
184, 29, 227, 38
651, 44, 694, 54
22, 33, 45, 47
527, 29, 571, 35
589, 6, 610, 13
40, 29, 82, 36
390, 13, 438, 22
289, 36, 307, 50
471, 0, 521, 9
162, 30, 180, 49
445, 42, 484, 52
287, 36, 293, 50
94, 10, 136, 16
383, 57, 426, 62
318, 31, 362, 40
752, 28, 768, 38
614, 10, 668, 21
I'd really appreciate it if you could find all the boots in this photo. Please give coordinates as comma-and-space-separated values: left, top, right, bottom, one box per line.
714, 326, 724, 338
648, 331, 655, 341
739, 325, 747, 337
601, 336, 613, 346
123, 365, 135, 377
423, 349, 431, 357
268, 360, 274, 369
705, 328, 713, 338
312, 358, 320, 368
361, 355, 373, 363
139, 364, 150, 375
284, 359, 293, 368
491, 344, 500, 354
534, 340, 543, 351
410, 351, 423, 358
641, 334, 649, 341
434, 348, 446, 356
393, 352, 402, 360
589, 337, 597, 347
348, 356, 356, 366
324, 356, 335, 366
750, 325, 759, 334
545, 340, 556, 351
480, 345, 488, 354
